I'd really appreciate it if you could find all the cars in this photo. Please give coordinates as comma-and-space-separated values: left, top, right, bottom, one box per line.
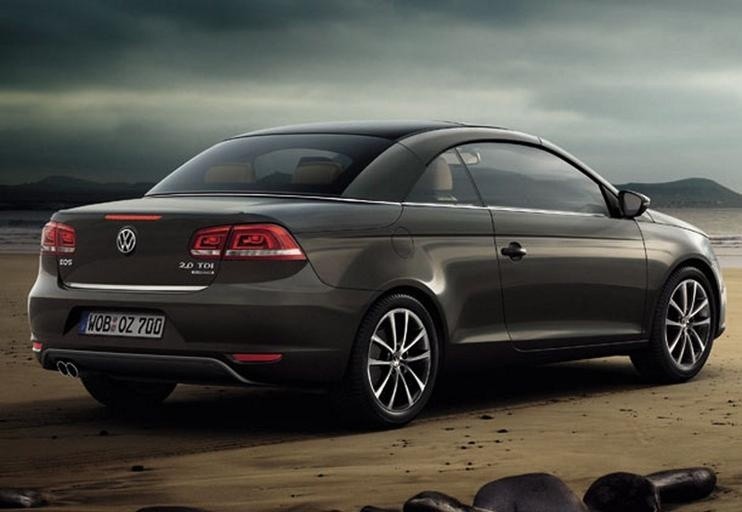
26, 118, 728, 428
